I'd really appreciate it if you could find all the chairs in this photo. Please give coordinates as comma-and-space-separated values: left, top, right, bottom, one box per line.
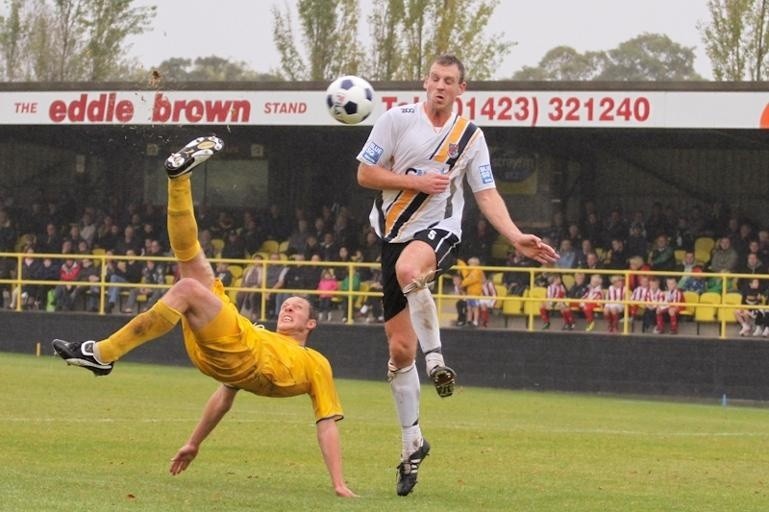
0, 235, 744, 338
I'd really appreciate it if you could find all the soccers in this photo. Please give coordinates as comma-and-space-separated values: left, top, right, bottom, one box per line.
325, 74, 375, 125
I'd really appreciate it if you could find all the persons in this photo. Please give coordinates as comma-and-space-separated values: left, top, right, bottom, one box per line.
1, 172, 768, 339
52, 134, 361, 500
356, 54, 563, 495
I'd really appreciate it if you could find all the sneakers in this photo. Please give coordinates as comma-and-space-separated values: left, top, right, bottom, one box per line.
52, 338, 114, 375
396, 437, 429, 496
163, 136, 225, 179
431, 365, 456, 398
